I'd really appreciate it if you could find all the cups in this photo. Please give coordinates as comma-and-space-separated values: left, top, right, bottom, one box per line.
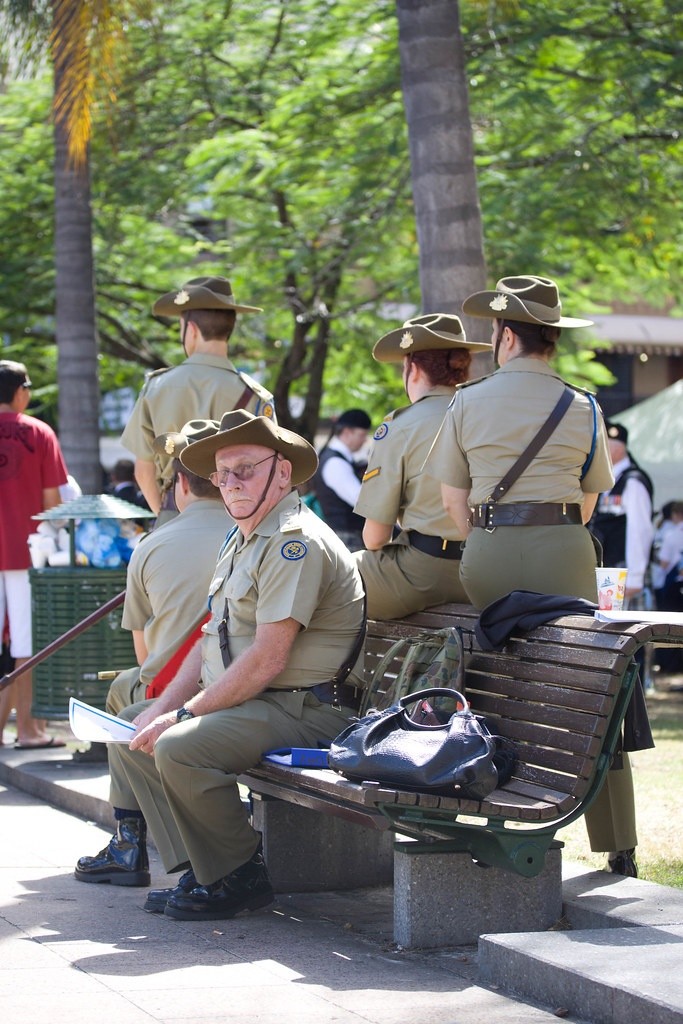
595, 565, 629, 612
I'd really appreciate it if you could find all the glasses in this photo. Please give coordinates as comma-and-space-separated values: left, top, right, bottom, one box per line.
208, 454, 283, 488
17, 377, 34, 392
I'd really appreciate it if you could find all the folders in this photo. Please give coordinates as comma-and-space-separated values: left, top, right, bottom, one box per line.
262, 747, 331, 770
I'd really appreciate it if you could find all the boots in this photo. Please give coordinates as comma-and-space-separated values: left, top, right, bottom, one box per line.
74, 817, 151, 887
163, 831, 275, 922
143, 863, 198, 911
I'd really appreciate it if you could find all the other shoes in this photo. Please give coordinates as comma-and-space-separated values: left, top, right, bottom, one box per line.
608, 848, 638, 877
74, 742, 110, 763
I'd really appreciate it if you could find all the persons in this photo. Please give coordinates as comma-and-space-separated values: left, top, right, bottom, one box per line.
116, 278, 280, 531
141, 408, 370, 920
418, 274, 643, 877
0, 361, 683, 749
73, 419, 238, 887
350, 312, 496, 619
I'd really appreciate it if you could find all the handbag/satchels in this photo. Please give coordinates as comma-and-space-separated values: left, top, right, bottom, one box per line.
329, 687, 516, 800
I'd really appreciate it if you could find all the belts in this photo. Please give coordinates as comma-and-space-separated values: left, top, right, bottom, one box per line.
467, 503, 582, 527
264, 680, 364, 713
404, 529, 466, 560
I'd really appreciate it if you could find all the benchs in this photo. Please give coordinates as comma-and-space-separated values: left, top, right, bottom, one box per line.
236, 604, 683, 952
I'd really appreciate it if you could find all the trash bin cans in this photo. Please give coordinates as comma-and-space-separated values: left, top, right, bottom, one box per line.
27, 494, 156, 722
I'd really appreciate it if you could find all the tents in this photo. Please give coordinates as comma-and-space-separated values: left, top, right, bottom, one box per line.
607, 378, 683, 512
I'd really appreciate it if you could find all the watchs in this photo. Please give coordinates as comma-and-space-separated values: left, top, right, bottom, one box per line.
177, 708, 195, 724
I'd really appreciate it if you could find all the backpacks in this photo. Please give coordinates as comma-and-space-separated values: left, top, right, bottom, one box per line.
358, 624, 475, 720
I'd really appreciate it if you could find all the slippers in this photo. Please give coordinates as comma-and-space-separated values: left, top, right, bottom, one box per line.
12, 733, 66, 750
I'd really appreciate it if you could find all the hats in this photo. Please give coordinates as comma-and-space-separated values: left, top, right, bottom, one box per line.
153, 274, 263, 315
151, 419, 220, 459
371, 311, 493, 364
337, 407, 373, 430
461, 272, 595, 328
603, 421, 628, 445
116, 459, 135, 476
181, 408, 319, 488
